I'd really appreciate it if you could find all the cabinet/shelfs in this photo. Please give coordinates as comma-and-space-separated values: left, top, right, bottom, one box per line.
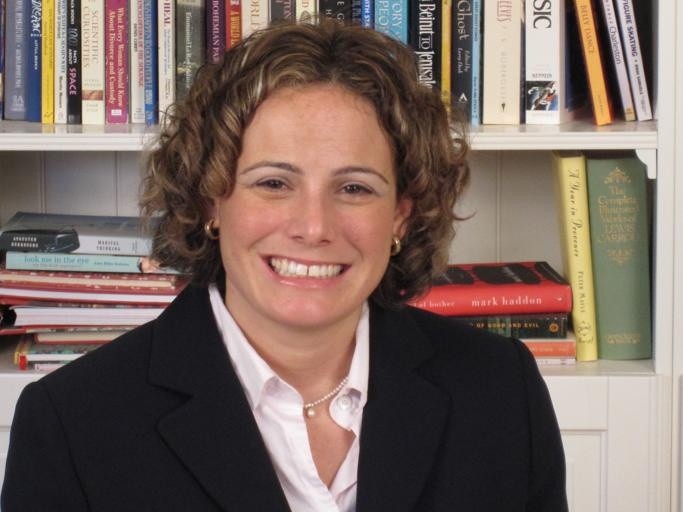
0, 0, 683, 512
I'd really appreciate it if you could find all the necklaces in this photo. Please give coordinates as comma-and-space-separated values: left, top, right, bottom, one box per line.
302, 373, 350, 418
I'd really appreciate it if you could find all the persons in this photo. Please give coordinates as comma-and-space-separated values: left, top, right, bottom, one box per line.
0, 14, 568, 512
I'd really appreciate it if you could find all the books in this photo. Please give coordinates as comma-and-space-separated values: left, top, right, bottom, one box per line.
1, 0, 653, 128
404, 150, 655, 365
2, 210, 192, 371
